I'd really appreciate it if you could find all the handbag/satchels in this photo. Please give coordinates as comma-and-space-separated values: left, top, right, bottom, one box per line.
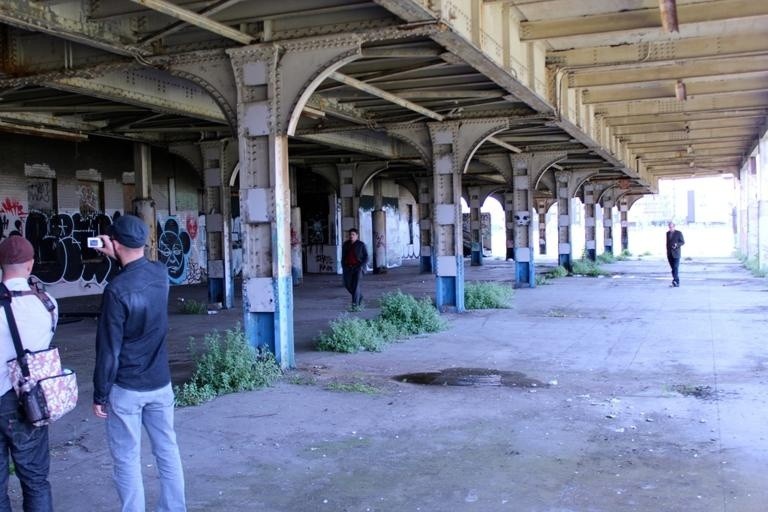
6, 346, 78, 428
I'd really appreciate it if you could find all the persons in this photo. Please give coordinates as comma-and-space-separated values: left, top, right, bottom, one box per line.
1, 235, 58, 511
666, 222, 684, 286
341, 228, 369, 308
93, 215, 188, 511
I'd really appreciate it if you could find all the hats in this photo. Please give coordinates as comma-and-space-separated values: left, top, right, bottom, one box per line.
104, 214, 149, 248
0, 235, 34, 265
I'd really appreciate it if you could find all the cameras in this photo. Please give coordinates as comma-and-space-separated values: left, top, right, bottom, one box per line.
88, 238, 103, 248
